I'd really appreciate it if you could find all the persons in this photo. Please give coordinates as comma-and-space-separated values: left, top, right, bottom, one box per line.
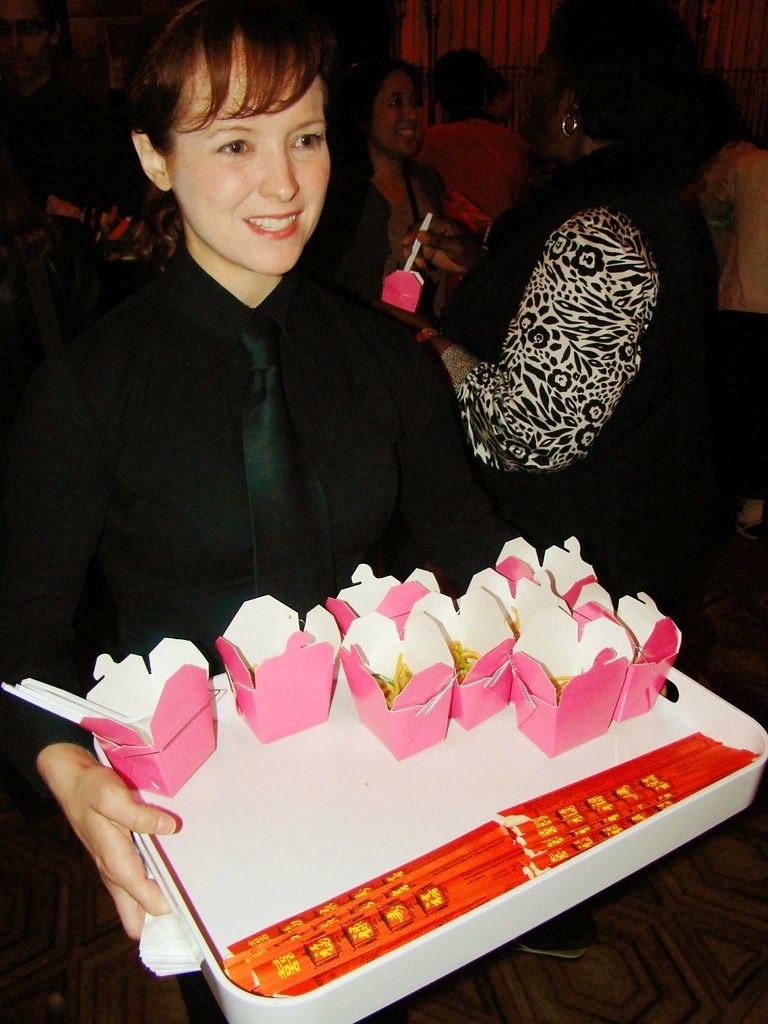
688, 73, 768, 540
307, 62, 448, 316
409, 48, 530, 237
375, 1, 719, 958
0, 0, 512, 1024
0, 0, 155, 366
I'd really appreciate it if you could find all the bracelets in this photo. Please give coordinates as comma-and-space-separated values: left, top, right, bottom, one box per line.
414, 327, 439, 343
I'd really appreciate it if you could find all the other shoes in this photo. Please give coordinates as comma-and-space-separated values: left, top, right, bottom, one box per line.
736, 517, 763, 540
509, 905, 596, 959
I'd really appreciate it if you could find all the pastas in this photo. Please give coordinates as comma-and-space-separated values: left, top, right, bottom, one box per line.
371, 607, 574, 710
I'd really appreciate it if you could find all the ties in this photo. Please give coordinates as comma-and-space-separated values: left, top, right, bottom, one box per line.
231, 317, 339, 615
